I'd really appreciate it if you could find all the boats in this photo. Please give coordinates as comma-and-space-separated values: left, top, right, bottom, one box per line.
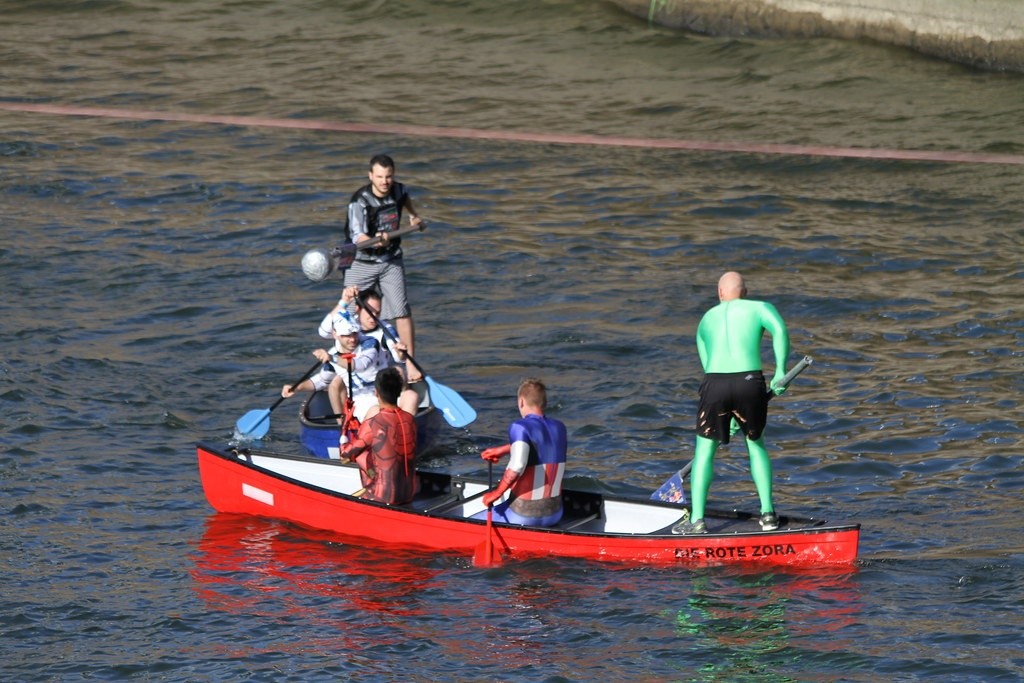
297, 387, 432, 464
195, 441, 862, 574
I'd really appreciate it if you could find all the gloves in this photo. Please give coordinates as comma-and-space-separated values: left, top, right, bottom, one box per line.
482, 490, 502, 507
481, 447, 504, 464
342, 396, 355, 433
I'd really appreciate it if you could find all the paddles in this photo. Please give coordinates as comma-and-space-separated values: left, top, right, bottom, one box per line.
650, 353, 813, 503
236, 360, 329, 440
353, 292, 478, 429
474, 453, 503, 564
341, 353, 358, 462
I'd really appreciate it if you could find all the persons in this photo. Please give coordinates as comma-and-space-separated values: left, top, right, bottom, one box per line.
343, 153, 423, 381
339, 367, 417, 507
318, 286, 408, 363
466, 379, 567, 527
280, 316, 419, 421
672, 271, 789, 535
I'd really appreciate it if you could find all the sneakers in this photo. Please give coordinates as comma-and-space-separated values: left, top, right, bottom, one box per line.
671, 511, 709, 534
758, 512, 780, 531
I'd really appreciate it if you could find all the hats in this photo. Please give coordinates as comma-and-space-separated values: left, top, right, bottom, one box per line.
333, 312, 361, 336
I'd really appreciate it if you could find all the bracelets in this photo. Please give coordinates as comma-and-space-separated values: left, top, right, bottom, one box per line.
339, 300, 347, 307
